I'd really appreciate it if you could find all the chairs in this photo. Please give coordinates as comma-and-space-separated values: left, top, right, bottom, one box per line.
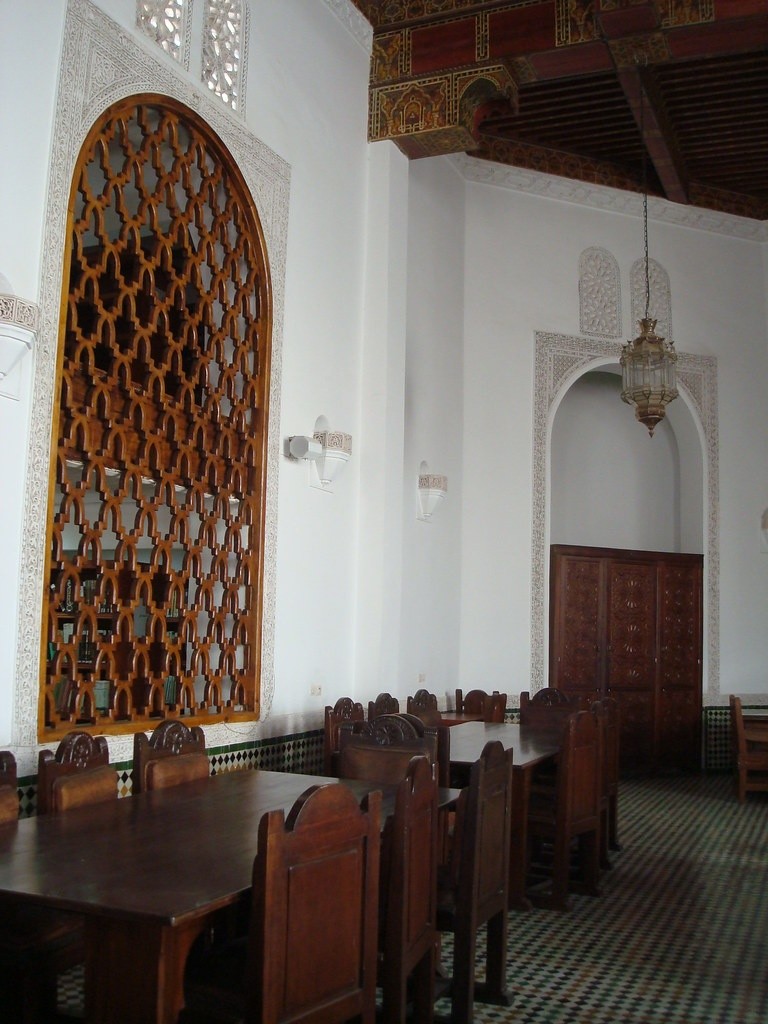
354, 713, 450, 786
368, 693, 400, 721
337, 713, 438, 781
730, 697, 768, 803
39, 731, 120, 813
188, 780, 386, 1024
133, 719, 211, 794
437, 742, 515, 1024
480, 692, 509, 722
406, 690, 440, 714
584, 696, 623, 869
454, 688, 499, 713
528, 709, 604, 912
0, 750, 20, 823
521, 687, 579, 724
373, 756, 443, 1024
323, 696, 365, 777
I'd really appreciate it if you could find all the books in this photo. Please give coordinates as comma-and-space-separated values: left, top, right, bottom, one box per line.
48, 577, 188, 715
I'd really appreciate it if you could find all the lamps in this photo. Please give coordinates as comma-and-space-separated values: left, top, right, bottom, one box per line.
615, 65, 679, 437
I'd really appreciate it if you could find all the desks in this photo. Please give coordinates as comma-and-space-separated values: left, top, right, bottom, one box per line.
449, 719, 565, 915
0, 764, 464, 1024
740, 708, 768, 776
441, 711, 492, 725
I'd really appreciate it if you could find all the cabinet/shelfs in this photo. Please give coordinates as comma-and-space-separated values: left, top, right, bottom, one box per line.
48, 560, 188, 722
550, 540, 707, 779
